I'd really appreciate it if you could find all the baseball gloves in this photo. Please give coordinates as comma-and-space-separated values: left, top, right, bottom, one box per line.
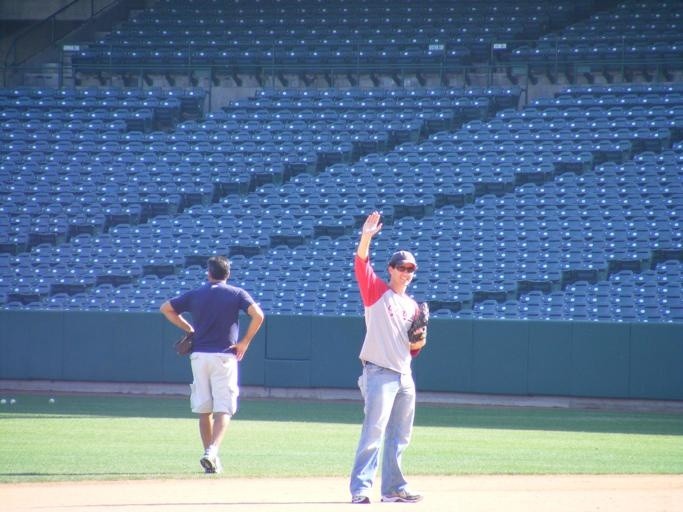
407, 302, 429, 342
175, 333, 192, 355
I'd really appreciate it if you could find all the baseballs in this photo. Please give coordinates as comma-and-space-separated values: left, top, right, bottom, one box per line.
10, 399, 15, 404
49, 398, 55, 404
1, 399, 6, 404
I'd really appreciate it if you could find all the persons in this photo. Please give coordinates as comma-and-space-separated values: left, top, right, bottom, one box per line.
346, 209, 429, 503
159, 255, 265, 474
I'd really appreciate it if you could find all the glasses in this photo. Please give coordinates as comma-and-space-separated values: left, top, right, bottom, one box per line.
395, 265, 415, 274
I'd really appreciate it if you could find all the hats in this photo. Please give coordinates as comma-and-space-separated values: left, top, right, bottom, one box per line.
388, 250, 417, 270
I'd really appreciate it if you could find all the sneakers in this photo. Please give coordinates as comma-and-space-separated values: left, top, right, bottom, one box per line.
379, 487, 422, 504
352, 492, 373, 505
198, 453, 222, 473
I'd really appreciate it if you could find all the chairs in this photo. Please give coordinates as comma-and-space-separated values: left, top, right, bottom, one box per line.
53, 1, 682, 82
1, 84, 683, 323
